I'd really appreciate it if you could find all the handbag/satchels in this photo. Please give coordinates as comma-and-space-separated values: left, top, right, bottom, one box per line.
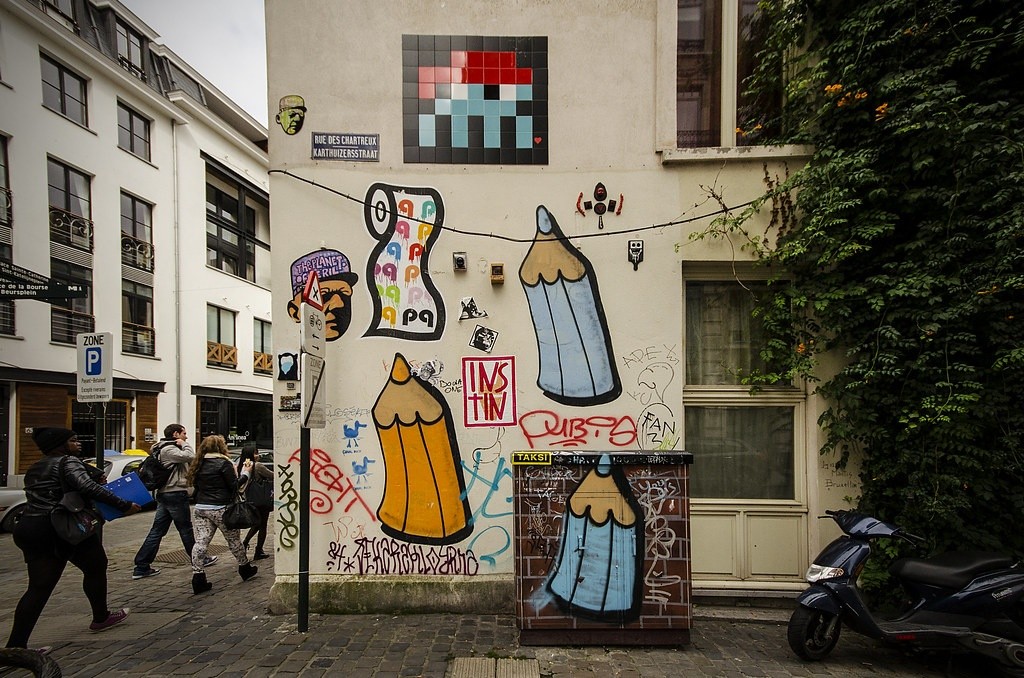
245, 465, 274, 511
51, 492, 106, 543
222, 492, 258, 530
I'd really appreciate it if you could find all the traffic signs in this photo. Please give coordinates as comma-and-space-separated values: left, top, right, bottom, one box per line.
0, 260, 88, 306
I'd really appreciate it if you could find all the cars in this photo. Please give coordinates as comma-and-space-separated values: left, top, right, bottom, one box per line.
81, 456, 159, 499
229, 447, 273, 469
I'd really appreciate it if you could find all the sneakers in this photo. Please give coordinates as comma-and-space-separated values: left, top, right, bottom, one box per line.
33, 645, 53, 656
89, 607, 132, 632
132, 569, 161, 579
192, 556, 218, 570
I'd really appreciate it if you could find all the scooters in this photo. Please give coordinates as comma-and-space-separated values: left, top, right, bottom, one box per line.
787, 509, 1024, 678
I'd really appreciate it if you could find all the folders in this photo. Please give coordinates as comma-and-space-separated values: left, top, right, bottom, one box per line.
95, 471, 154, 522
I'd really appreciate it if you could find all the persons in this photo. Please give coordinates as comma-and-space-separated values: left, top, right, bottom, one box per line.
4, 426, 132, 653
185, 433, 260, 595
132, 423, 218, 578
237, 446, 274, 559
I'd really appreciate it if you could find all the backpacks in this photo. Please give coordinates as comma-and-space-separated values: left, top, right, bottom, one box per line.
138, 441, 182, 490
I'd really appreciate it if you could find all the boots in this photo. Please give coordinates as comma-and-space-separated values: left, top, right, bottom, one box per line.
238, 563, 258, 582
254, 547, 270, 561
192, 573, 214, 595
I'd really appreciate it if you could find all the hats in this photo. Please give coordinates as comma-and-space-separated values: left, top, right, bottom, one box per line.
32, 426, 78, 455
